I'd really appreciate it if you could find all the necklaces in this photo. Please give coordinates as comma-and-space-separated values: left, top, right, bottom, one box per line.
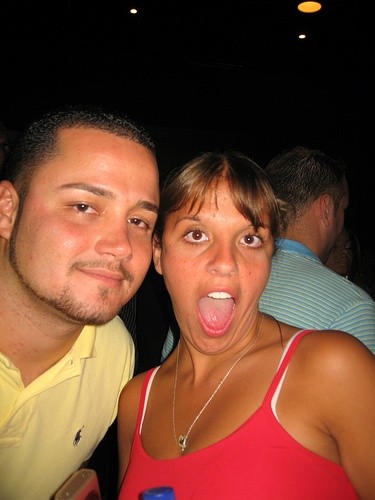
171, 313, 264, 452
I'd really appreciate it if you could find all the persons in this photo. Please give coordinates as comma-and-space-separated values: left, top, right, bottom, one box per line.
323, 222, 361, 282
0, 106, 159, 500
116, 147, 375, 500
258, 143, 375, 360
